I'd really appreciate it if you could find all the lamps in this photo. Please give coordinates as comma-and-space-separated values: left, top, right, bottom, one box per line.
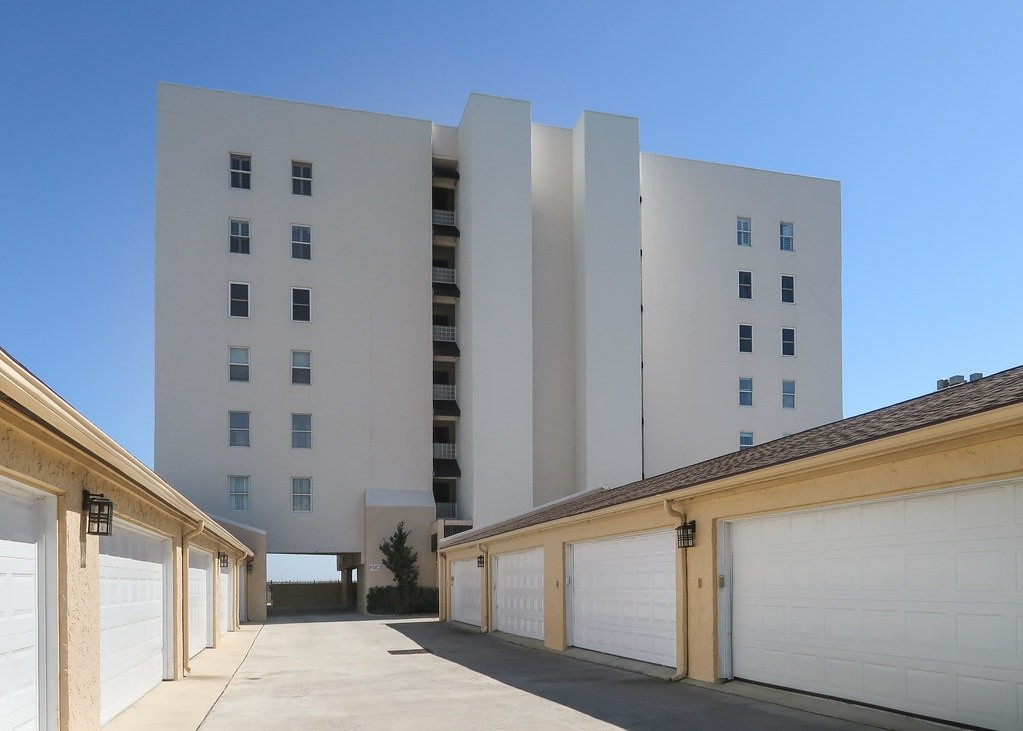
218, 551, 228, 567
478, 555, 484, 567
247, 564, 253, 573
82, 489, 114, 536
675, 520, 696, 548
